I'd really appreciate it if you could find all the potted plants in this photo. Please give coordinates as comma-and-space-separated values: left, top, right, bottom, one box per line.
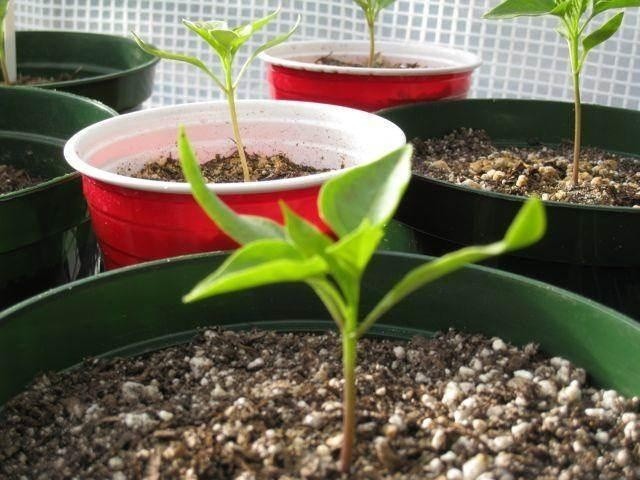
0, 0, 161, 116
258, 0, 483, 114
372, 0, 640, 322
0, 122, 636, 480
62, 8, 406, 273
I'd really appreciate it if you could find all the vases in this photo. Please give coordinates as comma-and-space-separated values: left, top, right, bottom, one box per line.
1, 86, 120, 312
